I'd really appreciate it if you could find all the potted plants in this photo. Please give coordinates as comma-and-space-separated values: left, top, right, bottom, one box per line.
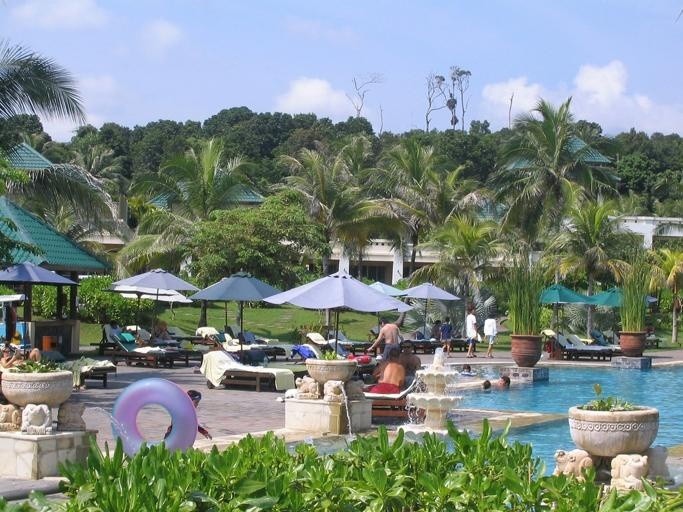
618, 251, 653, 358
504, 250, 548, 366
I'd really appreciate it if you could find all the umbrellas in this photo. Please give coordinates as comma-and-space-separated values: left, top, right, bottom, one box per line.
0, 260, 78, 359
102, 286, 179, 332
538, 283, 598, 348
263, 272, 414, 360
112, 267, 199, 321
369, 282, 408, 334
591, 286, 658, 346
187, 271, 282, 363
401, 282, 460, 339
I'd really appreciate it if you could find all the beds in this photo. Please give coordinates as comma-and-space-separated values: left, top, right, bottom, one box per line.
210, 333, 267, 362
111, 331, 183, 368
0, 344, 27, 373
163, 327, 203, 344
416, 340, 435, 354
327, 329, 375, 353
567, 334, 619, 361
194, 325, 219, 342
540, 328, 557, 342
299, 330, 354, 355
368, 328, 415, 353
202, 351, 293, 391
416, 326, 466, 351
40, 346, 115, 388
224, 322, 294, 355
551, 335, 613, 362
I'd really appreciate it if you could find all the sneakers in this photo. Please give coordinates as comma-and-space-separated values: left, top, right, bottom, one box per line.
467, 355, 473, 357
466, 354, 473, 358
447, 355, 451, 357
472, 355, 477, 358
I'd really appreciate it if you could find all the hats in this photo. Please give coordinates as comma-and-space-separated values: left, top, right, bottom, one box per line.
466, 305, 478, 310
432, 320, 441, 324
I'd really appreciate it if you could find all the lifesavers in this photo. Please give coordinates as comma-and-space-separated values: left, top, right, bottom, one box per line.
461, 370, 477, 376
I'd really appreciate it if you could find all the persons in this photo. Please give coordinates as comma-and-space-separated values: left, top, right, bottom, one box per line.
150, 321, 171, 342
363, 348, 405, 394
110, 320, 131, 342
499, 376, 511, 386
163, 390, 212, 441
461, 364, 474, 374
400, 341, 421, 389
441, 317, 453, 357
466, 305, 478, 358
482, 380, 492, 391
367, 318, 400, 360
0, 344, 41, 367
484, 312, 497, 358
431, 320, 441, 339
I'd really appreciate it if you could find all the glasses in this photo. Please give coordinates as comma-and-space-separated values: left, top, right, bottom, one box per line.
403, 346, 411, 348
